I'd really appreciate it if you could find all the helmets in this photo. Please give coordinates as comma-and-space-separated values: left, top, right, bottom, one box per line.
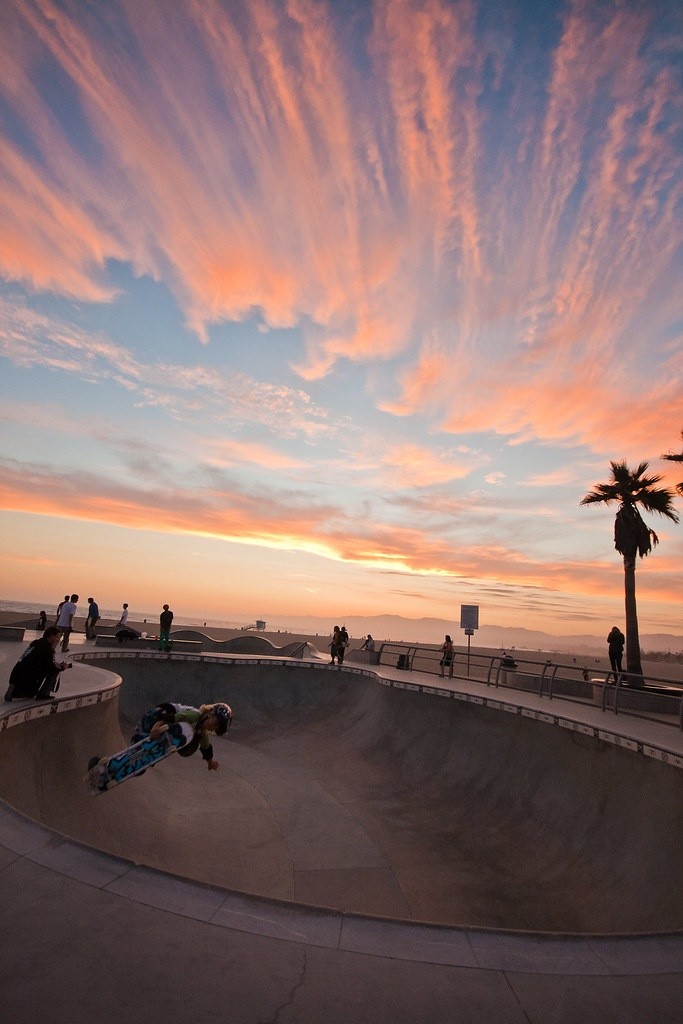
214, 703, 232, 736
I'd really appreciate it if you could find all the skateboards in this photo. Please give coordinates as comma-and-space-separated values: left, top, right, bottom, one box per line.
82, 719, 197, 799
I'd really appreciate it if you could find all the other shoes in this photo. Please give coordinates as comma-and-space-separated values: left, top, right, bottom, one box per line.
4, 684, 16, 702
439, 674, 443, 677
328, 662, 335, 664
35, 694, 55, 700
62, 649, 69, 652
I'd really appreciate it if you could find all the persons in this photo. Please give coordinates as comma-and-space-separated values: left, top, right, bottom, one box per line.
606, 626, 625, 682
159, 604, 173, 652
116, 603, 128, 626
88, 700, 235, 776
327, 625, 349, 665
438, 634, 454, 679
360, 635, 375, 651
57, 593, 79, 652
2, 625, 69, 702
35, 610, 47, 630
84, 597, 99, 639
